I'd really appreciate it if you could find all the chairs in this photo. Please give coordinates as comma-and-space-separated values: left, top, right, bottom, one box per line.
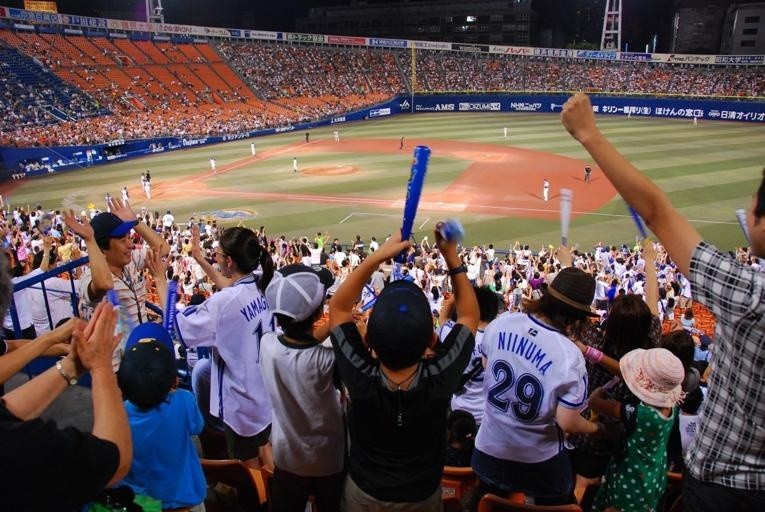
1, 28, 765, 512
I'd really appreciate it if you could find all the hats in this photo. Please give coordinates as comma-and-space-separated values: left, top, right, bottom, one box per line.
118, 322, 178, 412
265, 263, 336, 325
536, 266, 601, 320
366, 279, 434, 371
88, 212, 139, 241
618, 347, 686, 408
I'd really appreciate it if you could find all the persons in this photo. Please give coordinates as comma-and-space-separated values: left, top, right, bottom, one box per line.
209, 156, 216, 171
251, 142, 255, 156
585, 164, 591, 182
1, 187, 761, 511
141, 170, 152, 200
397, 47, 764, 104
293, 158, 297, 172
1, 28, 409, 148
543, 179, 550, 201
503, 126, 507, 137
559, 93, 765, 511
